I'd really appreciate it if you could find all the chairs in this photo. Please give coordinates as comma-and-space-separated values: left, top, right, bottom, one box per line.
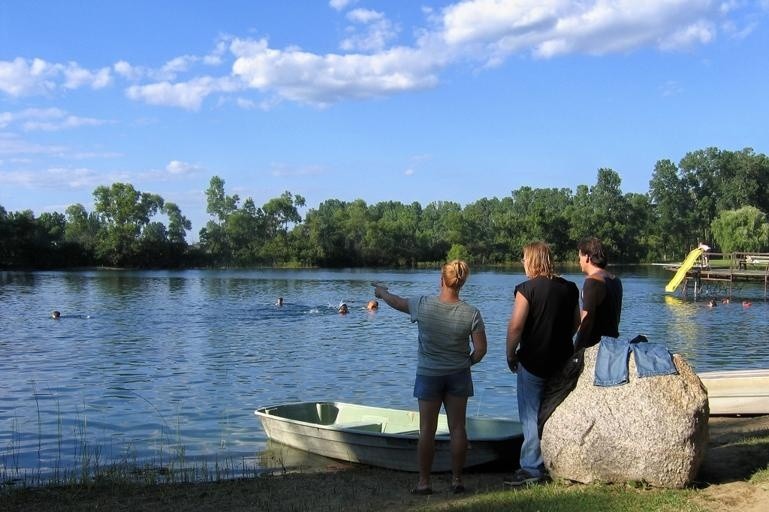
504, 469, 541, 485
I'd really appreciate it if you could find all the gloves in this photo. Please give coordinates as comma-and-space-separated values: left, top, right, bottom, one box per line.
697, 367, 769, 417
254, 401, 525, 472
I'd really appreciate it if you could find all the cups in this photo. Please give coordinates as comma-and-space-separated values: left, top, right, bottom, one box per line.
521, 259, 527, 263
372, 306, 376, 308
340, 306, 347, 310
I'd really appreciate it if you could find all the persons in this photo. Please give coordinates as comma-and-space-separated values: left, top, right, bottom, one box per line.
368, 299, 379, 311
276, 297, 285, 307
720, 298, 729, 304
706, 300, 717, 309
503, 240, 584, 488
338, 303, 350, 316
372, 258, 489, 495
741, 297, 752, 308
574, 236, 624, 354
51, 311, 61, 319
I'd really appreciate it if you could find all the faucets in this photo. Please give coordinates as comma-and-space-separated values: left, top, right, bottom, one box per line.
452, 485, 464, 493
408, 486, 432, 495
